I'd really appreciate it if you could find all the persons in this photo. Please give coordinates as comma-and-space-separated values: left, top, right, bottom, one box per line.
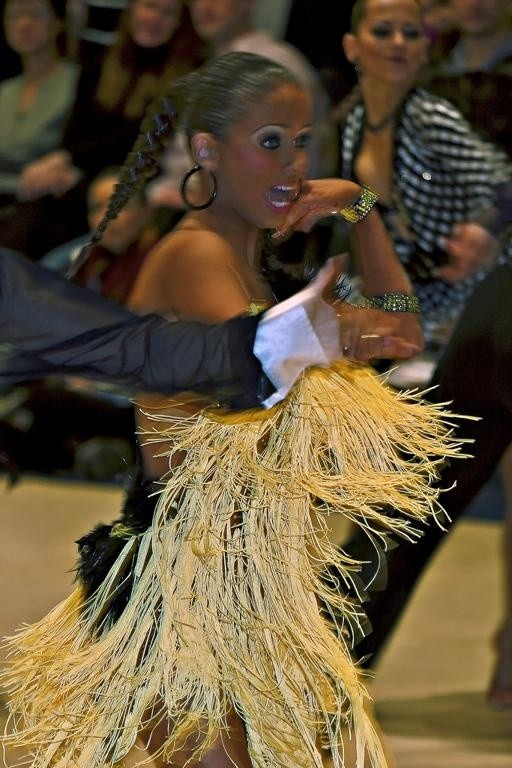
68, 51, 426, 765
310, 252, 512, 705
0, 2, 511, 525
